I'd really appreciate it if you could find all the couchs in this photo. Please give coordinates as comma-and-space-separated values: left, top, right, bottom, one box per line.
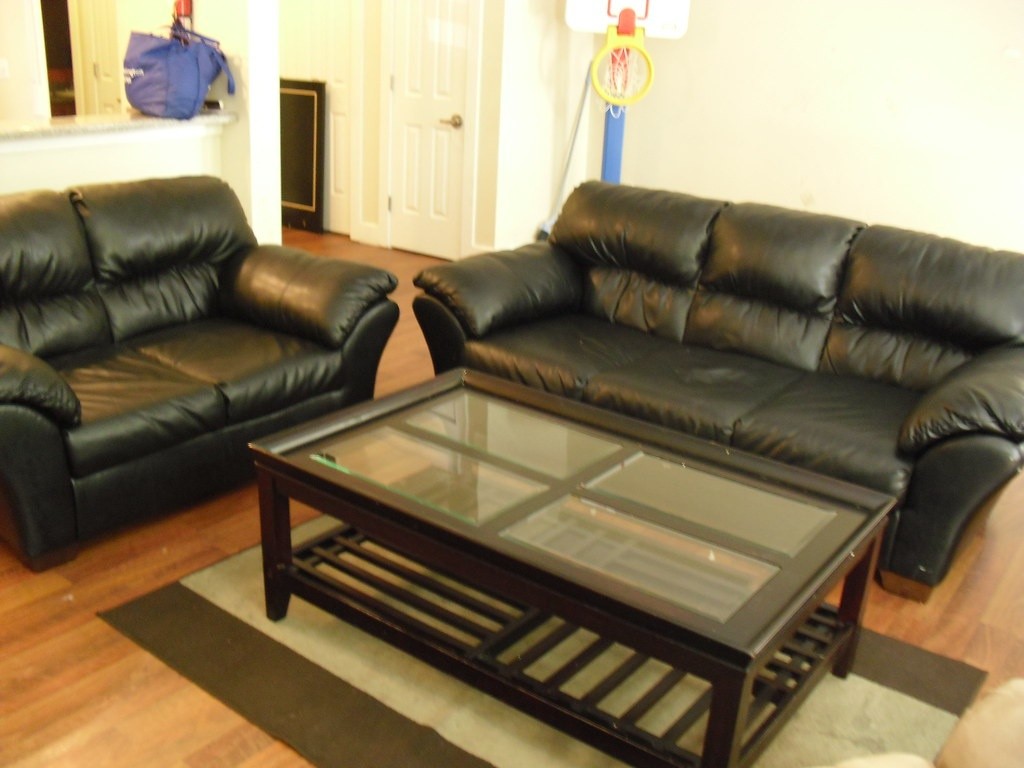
410, 179, 1024, 606
0, 174, 401, 576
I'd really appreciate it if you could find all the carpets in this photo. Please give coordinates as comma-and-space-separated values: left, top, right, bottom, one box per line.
94, 512, 989, 768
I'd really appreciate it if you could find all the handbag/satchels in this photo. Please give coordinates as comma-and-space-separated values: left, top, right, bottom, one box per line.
123, 18, 236, 121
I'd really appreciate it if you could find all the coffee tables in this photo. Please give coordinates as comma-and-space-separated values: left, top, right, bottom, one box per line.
245, 367, 897, 768
